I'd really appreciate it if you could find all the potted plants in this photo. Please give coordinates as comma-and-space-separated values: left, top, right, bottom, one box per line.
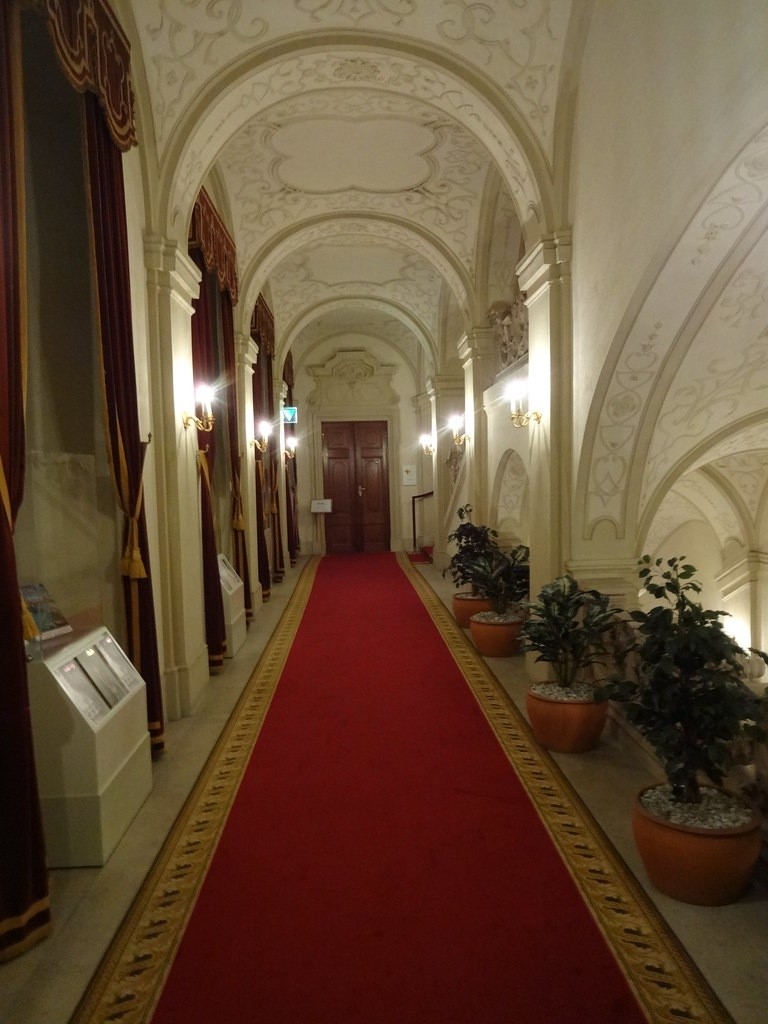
461, 526, 531, 657
622, 553, 768, 906
514, 573, 640, 756
443, 502, 496, 627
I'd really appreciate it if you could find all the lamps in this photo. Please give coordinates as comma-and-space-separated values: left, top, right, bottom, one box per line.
453, 417, 471, 446
251, 422, 269, 453
421, 437, 435, 455
283, 437, 298, 458
182, 384, 216, 432
509, 382, 543, 428
